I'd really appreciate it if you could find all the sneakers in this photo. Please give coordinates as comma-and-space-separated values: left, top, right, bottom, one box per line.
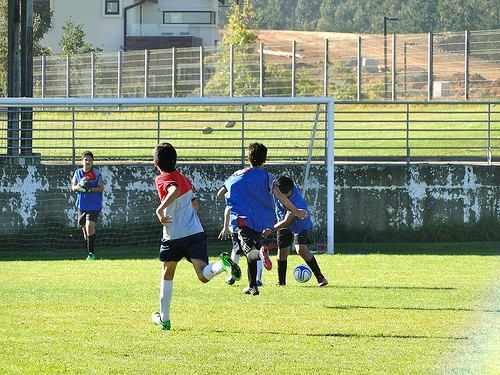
220, 251, 242, 281
259, 245, 272, 270
256, 280, 262, 286
225, 276, 235, 285
243, 284, 259, 295
152, 311, 171, 330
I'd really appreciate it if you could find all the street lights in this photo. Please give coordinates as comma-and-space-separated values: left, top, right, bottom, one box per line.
384, 15, 399, 98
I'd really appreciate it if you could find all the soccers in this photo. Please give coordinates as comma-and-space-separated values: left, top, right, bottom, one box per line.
293, 264, 312, 283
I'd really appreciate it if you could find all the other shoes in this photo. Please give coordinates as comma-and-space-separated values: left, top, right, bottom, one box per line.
86, 252, 95, 260
317, 278, 328, 286
275, 282, 281, 286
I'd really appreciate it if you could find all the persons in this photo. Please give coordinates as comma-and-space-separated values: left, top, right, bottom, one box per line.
72, 151, 105, 259
152, 142, 242, 330
216, 143, 328, 294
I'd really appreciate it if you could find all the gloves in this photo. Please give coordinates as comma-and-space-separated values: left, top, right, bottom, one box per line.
78, 176, 88, 187
76, 186, 92, 192
